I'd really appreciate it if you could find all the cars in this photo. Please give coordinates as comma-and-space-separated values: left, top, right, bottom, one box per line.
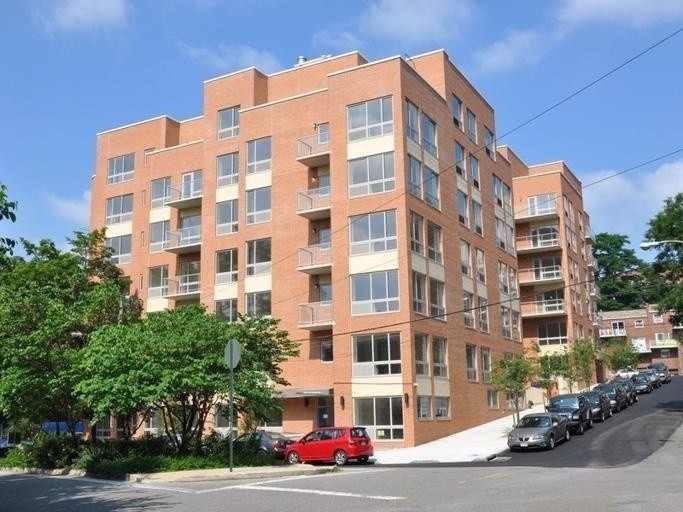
233, 430, 296, 459
153, 434, 208, 453
507, 363, 671, 452
0, 440, 35, 457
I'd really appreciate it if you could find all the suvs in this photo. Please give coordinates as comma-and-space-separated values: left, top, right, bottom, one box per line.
286, 428, 376, 466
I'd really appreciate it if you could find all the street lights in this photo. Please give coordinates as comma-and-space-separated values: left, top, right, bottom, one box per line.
639, 238, 682, 251
